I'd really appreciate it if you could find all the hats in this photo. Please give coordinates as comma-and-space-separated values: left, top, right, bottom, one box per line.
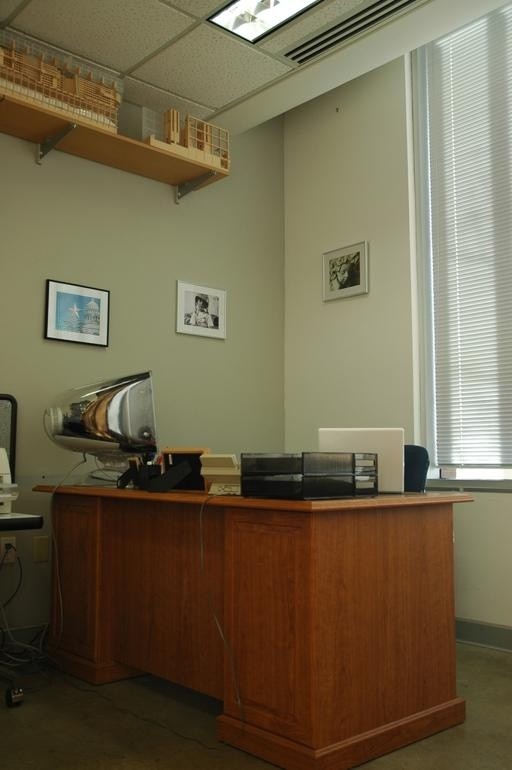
196, 294, 209, 308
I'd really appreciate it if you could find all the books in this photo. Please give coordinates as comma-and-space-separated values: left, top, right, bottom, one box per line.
356, 482, 374, 489
355, 475, 370, 480
355, 459, 374, 467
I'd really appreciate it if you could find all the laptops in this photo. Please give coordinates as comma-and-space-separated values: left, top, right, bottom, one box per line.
316, 424, 408, 495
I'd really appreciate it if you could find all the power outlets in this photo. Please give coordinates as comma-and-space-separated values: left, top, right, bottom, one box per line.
1, 537, 16, 563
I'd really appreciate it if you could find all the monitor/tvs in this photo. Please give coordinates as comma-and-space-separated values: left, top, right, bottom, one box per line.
43, 372, 158, 480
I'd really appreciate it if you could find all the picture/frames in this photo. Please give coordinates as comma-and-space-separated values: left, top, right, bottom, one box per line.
321, 241, 370, 303
44, 275, 110, 350
175, 281, 229, 341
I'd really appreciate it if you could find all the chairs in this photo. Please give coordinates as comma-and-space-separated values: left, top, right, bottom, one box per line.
404, 444, 430, 492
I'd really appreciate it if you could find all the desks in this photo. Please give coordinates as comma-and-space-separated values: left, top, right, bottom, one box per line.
0, 513, 43, 532
32, 481, 475, 768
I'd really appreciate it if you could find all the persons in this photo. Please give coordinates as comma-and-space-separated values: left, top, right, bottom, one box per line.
329, 252, 360, 291
184, 293, 218, 329
